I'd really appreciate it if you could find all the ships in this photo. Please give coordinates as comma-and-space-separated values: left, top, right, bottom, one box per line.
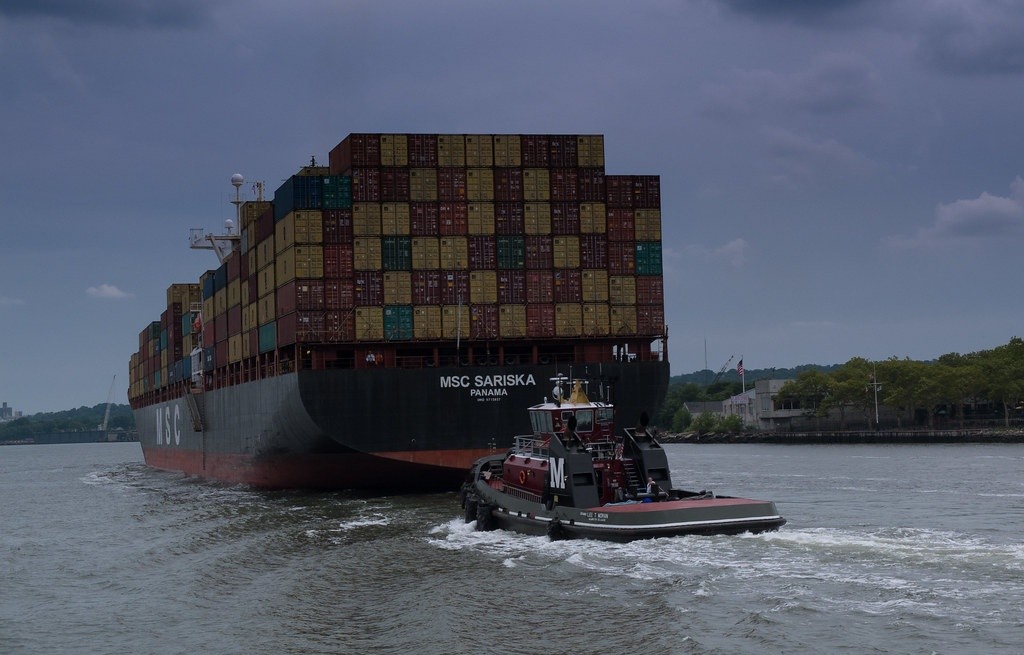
127, 132, 671, 488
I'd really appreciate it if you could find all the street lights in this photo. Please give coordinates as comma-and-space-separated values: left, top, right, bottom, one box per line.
866, 358, 883, 431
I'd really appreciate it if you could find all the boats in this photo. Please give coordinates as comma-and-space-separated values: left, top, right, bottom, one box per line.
461, 364, 788, 541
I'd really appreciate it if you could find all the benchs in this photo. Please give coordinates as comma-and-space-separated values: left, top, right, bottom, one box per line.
626, 491, 667, 501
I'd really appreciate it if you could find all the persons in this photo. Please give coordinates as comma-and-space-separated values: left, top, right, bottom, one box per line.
375, 352, 383, 365
366, 350, 375, 366
647, 477, 656, 494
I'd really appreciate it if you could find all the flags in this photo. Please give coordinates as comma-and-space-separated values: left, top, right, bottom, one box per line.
737, 360, 743, 376
